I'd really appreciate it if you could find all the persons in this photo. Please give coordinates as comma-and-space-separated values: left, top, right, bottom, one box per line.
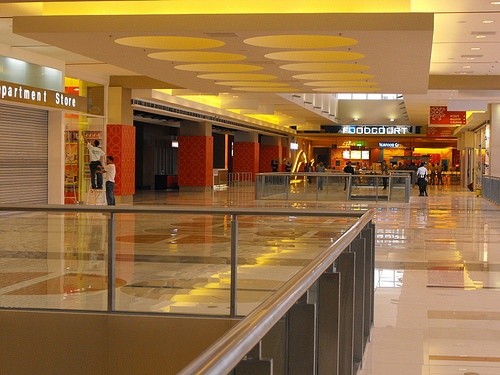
285, 161, 292, 172
83, 132, 106, 189
271, 159, 279, 172
428, 165, 443, 185
391, 162, 419, 184
381, 160, 389, 189
96, 156, 116, 205
355, 162, 360, 170
417, 163, 428, 196
343, 162, 354, 191
304, 161, 325, 190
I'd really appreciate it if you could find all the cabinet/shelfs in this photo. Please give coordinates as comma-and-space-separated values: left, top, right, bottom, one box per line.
64, 130, 104, 204
